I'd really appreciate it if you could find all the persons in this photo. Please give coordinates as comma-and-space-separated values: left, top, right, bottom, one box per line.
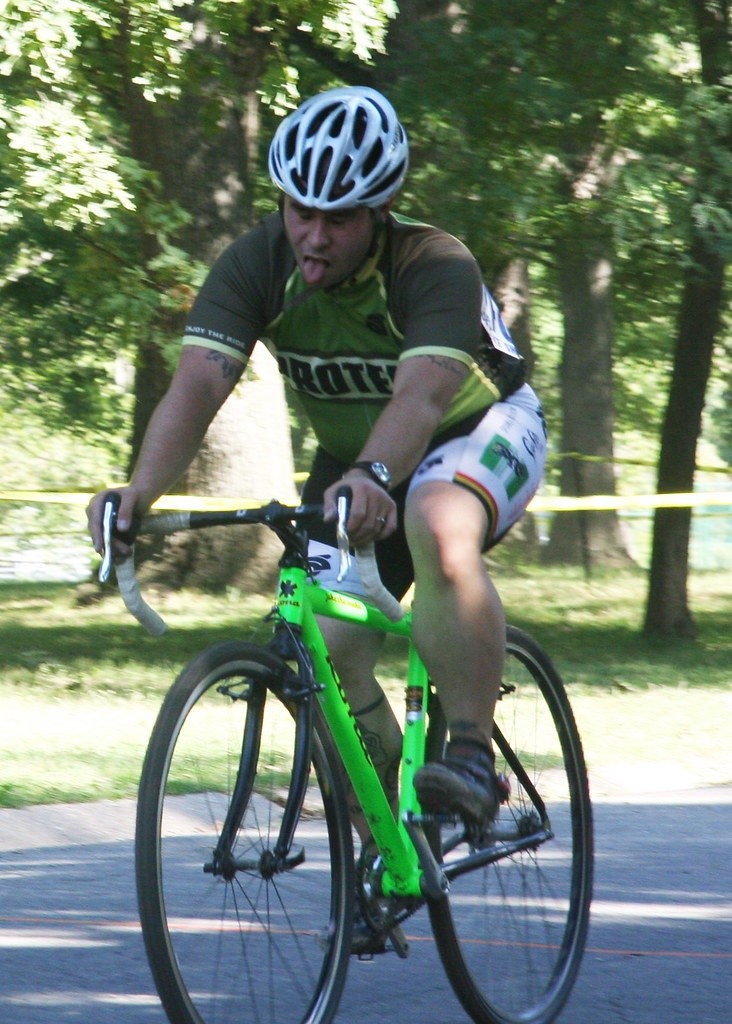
84, 85, 550, 951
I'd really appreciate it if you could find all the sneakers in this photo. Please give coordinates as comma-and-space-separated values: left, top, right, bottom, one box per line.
413, 741, 498, 822
350, 842, 400, 953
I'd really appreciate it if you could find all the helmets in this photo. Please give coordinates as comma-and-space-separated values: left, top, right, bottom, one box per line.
268, 86, 409, 210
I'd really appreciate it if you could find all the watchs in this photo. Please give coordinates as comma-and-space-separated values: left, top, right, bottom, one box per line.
346, 459, 393, 491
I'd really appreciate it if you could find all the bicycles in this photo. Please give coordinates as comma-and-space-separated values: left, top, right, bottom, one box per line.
98, 486, 595, 1024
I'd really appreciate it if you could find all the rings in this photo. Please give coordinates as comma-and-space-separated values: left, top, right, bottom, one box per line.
375, 516, 385, 524
